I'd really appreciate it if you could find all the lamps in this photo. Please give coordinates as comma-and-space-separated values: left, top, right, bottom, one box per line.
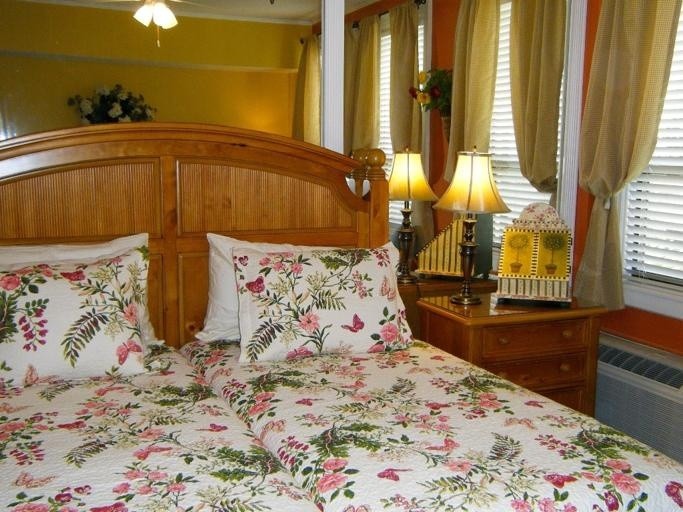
432, 147, 511, 305
389, 148, 439, 284
133, 1, 177, 47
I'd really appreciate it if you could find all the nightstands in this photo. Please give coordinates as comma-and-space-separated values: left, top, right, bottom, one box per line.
399, 273, 495, 336
417, 293, 609, 417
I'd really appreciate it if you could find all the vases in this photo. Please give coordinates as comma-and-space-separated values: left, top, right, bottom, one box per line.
442, 115, 450, 141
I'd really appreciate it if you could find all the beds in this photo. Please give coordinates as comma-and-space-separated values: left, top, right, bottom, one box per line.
1, 121, 683, 512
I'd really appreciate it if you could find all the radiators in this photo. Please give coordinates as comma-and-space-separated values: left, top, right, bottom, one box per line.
597, 336, 683, 463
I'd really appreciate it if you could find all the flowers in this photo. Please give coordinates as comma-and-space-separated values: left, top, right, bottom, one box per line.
417, 69, 450, 113
67, 83, 159, 125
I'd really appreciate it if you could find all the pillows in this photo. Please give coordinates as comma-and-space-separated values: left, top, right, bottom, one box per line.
0, 232, 166, 347
0, 244, 150, 384
233, 245, 412, 363
195, 232, 400, 342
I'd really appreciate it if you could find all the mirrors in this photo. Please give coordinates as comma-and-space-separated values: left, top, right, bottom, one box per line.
0, 0, 431, 293
